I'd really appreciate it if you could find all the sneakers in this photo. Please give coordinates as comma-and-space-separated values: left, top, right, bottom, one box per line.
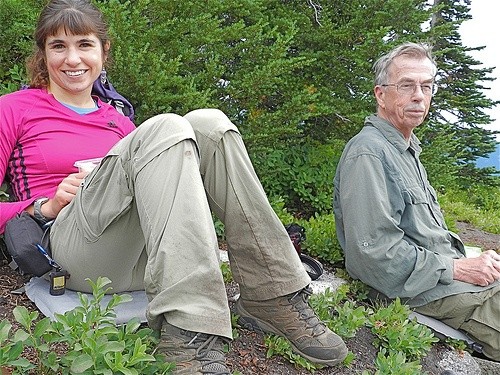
159, 320, 228, 375
235, 287, 348, 367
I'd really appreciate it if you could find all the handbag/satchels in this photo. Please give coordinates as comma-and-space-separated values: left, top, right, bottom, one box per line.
5, 209, 53, 278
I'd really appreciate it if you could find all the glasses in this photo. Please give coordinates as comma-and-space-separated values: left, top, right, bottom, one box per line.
381, 81, 438, 95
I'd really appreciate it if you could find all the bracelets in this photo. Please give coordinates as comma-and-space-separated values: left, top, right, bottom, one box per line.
34, 197, 50, 224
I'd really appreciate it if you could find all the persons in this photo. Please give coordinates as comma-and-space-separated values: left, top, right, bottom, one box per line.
0, 0, 348, 375
335, 40, 500, 362
